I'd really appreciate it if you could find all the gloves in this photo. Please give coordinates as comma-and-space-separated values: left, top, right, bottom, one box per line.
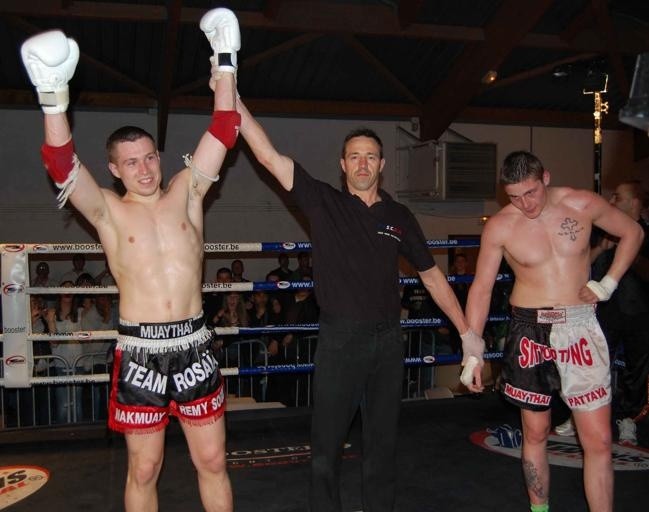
20, 28, 79, 115
199, 7, 241, 71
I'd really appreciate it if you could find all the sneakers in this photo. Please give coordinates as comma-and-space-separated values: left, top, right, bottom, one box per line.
554, 416, 577, 436
618, 417, 638, 446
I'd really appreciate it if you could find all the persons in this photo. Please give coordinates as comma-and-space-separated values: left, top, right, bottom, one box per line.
552, 179, 649, 447
460, 150, 646, 512
18, 7, 241, 512
203, 252, 321, 408
29, 253, 120, 417
209, 55, 486, 512
398, 253, 476, 399
